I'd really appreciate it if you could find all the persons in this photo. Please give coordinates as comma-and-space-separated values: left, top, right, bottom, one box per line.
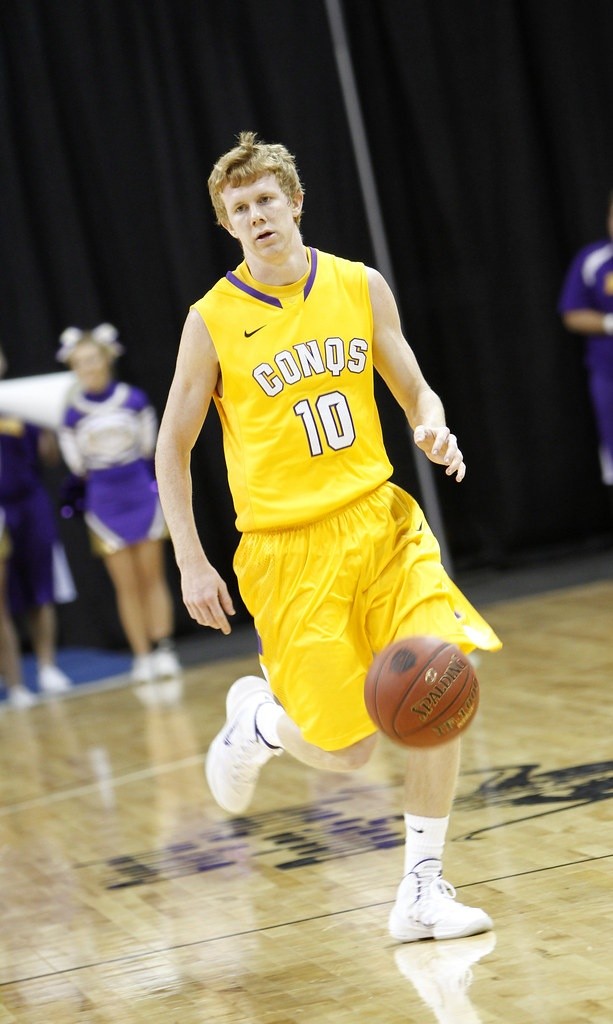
0, 324, 182, 705
154, 132, 504, 943
560, 201, 613, 486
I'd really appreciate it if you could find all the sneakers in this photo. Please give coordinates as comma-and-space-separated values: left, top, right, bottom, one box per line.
395, 928, 495, 991
388, 870, 490, 944
205, 676, 285, 814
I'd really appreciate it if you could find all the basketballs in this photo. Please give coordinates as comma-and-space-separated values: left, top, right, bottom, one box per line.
364, 635, 482, 751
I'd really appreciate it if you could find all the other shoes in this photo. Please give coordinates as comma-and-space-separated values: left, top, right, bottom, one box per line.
4, 685, 35, 708
38, 667, 67, 691
131, 648, 181, 683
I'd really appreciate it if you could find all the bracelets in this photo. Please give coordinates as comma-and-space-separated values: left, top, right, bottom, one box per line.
602, 312, 613, 335
